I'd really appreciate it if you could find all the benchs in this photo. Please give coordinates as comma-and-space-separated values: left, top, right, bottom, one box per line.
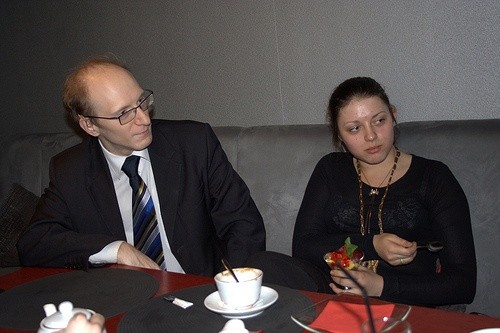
0, 118, 499, 318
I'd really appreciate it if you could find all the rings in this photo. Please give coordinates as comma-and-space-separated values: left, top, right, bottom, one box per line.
400, 258, 405, 265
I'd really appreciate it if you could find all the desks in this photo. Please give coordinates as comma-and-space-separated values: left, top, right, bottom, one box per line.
0, 266, 500, 333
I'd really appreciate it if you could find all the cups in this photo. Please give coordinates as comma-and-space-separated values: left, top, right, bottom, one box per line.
214, 268, 264, 308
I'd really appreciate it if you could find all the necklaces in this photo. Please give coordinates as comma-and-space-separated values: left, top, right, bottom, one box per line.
361, 167, 392, 235
355, 144, 401, 237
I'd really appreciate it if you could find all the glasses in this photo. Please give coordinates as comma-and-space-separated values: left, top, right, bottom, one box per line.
84, 88, 154, 125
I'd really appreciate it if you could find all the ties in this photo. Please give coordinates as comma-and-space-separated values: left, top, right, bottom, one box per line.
121, 155, 167, 272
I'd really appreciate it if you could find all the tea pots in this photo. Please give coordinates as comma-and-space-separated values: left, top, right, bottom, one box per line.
37, 302, 92, 333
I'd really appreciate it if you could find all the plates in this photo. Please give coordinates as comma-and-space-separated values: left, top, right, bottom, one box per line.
204, 284, 279, 321
291, 292, 412, 333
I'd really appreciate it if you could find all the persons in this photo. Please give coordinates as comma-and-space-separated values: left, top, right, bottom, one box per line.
54, 312, 108, 333
228, 77, 478, 312
15, 51, 266, 277
